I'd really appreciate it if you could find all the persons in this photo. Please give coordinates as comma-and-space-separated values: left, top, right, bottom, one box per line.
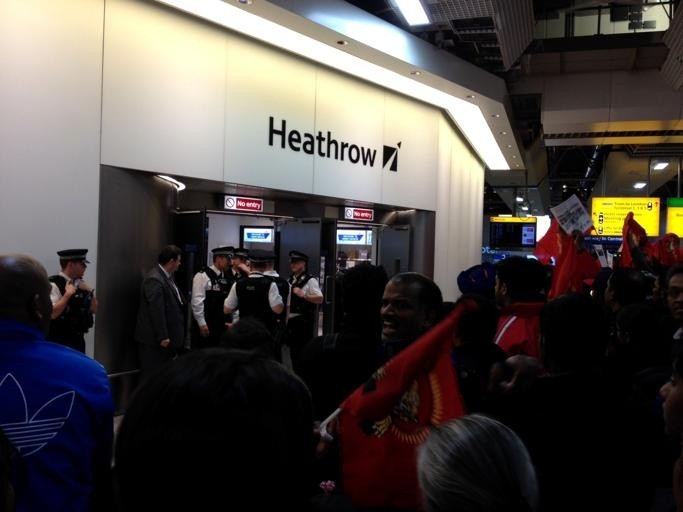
43, 248, 99, 355
0, 252, 117, 512
114, 231, 683, 511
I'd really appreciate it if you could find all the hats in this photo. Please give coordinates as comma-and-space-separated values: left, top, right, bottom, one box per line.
287, 250, 308, 264
248, 249, 270, 263
234, 248, 250, 260
56, 249, 90, 264
211, 246, 235, 255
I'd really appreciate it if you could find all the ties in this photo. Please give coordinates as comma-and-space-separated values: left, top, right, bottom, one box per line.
168, 276, 183, 305
